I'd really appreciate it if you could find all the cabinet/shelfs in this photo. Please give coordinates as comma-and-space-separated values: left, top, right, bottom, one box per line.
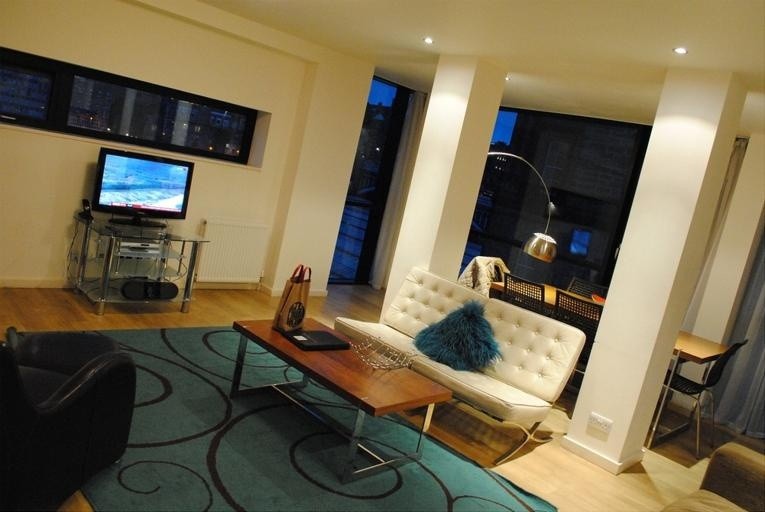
73, 214, 212, 316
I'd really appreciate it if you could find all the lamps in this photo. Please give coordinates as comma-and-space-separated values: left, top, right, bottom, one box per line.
482, 149, 563, 261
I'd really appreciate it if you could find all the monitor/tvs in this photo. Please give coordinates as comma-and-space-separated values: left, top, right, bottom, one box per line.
91, 147, 194, 229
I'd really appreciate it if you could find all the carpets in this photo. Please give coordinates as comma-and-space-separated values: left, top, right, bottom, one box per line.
79, 326, 552, 511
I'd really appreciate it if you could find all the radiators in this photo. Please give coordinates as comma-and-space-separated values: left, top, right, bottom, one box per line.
190, 216, 272, 285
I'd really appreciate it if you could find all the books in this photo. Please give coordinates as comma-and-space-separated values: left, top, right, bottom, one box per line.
280, 329, 351, 351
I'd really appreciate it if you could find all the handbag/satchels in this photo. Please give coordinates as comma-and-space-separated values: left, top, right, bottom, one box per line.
272, 265, 312, 334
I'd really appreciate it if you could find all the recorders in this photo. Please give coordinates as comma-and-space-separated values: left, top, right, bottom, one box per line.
120, 281, 178, 301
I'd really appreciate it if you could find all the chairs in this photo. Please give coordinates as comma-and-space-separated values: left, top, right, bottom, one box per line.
0, 325, 137, 511
